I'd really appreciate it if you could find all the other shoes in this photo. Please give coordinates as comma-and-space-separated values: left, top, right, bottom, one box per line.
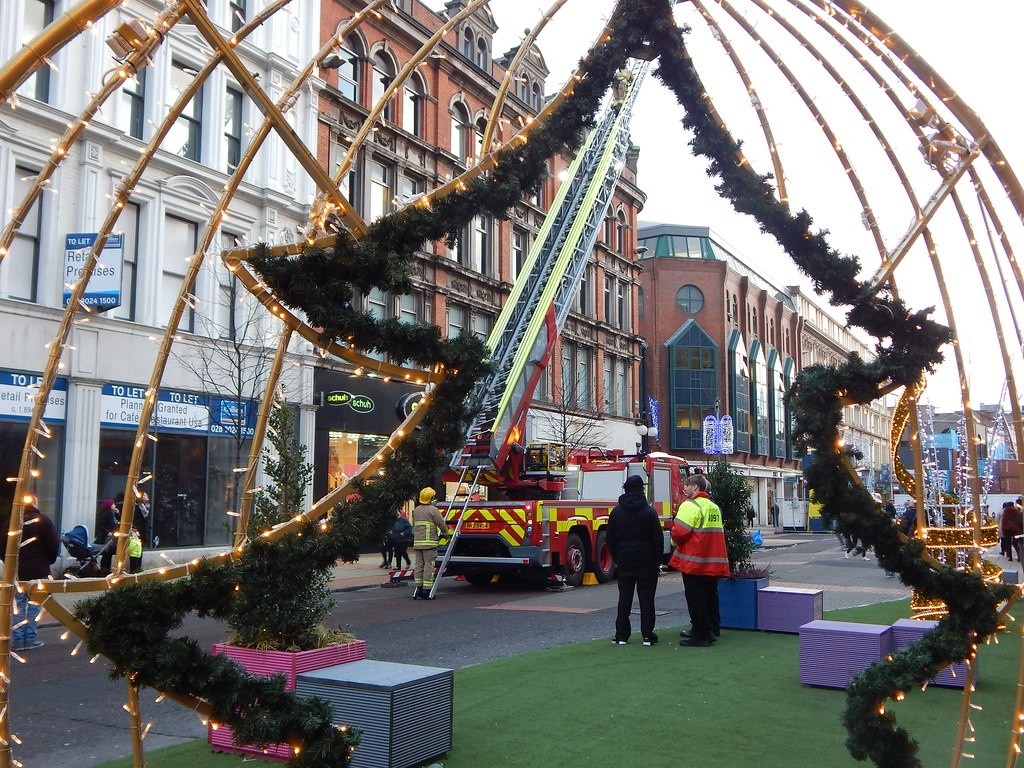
379, 560, 388, 568
386, 563, 391, 569
710, 631, 716, 643
845, 551, 849, 558
456, 575, 465, 581
413, 586, 436, 599
862, 557, 870, 561
393, 567, 401, 570
405, 564, 410, 569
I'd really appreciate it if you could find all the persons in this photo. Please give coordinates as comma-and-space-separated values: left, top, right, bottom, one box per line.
605, 474, 665, 646
832, 498, 929, 579
0, 495, 61, 652
747, 504, 755, 528
96, 490, 150, 577
992, 498, 1024, 562
411, 486, 449, 600
770, 503, 780, 527
668, 476, 731, 647
378, 512, 414, 570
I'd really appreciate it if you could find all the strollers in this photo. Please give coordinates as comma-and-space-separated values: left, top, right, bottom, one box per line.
60, 524, 114, 578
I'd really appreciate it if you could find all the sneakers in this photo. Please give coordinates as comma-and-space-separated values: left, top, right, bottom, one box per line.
643, 632, 659, 646
11, 639, 25, 651
24, 638, 44, 649
611, 637, 627, 645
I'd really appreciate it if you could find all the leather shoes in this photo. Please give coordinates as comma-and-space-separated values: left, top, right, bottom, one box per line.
680, 630, 693, 638
679, 639, 709, 647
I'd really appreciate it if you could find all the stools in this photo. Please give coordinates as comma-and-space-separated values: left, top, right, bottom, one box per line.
297, 659, 454, 768
798, 618, 978, 688
758, 586, 823, 633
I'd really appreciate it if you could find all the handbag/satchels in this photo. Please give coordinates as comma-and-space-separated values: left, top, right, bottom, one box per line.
408, 533, 414, 547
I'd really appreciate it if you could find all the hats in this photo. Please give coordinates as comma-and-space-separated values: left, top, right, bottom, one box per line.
101, 499, 114, 509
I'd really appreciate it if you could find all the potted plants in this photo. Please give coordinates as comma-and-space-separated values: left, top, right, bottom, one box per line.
208, 406, 366, 762
701, 453, 783, 629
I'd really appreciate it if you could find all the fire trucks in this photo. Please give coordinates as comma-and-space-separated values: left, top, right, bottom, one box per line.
388, 47, 704, 592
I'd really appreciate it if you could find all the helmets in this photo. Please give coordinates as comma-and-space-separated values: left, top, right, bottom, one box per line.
418, 487, 436, 505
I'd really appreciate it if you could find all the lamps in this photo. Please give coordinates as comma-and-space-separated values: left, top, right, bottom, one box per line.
635, 246, 649, 253
322, 54, 346, 70
